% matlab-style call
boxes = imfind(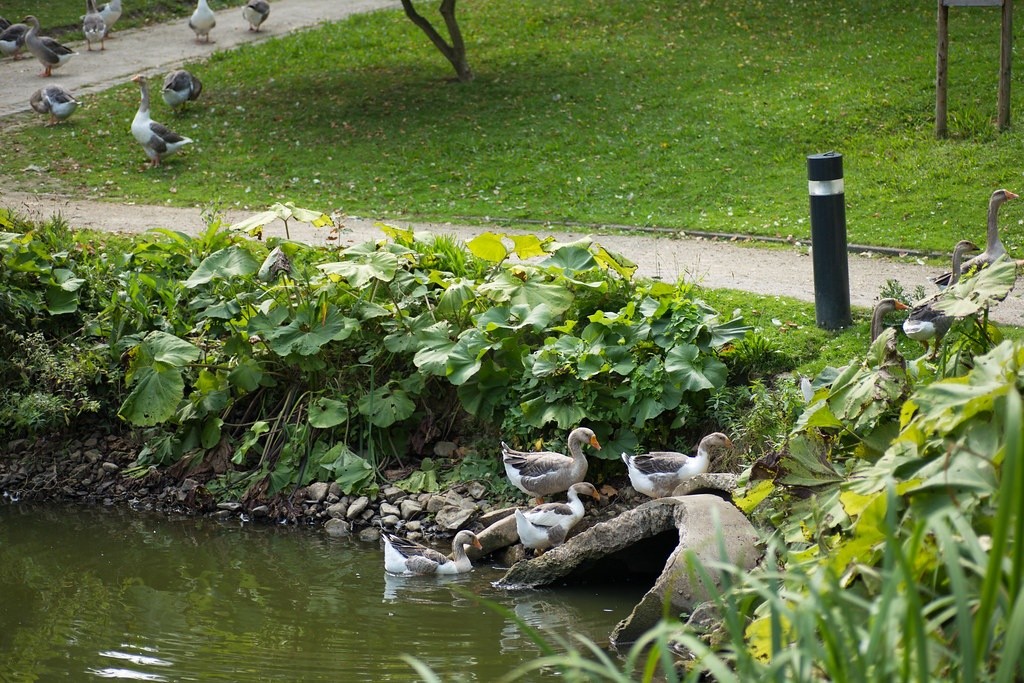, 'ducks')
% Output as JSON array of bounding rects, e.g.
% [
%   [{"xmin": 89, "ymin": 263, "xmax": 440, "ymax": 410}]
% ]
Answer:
[
  {"xmin": 904, "ymin": 239, "xmax": 981, "ymax": 360},
  {"xmin": 160, "ymin": 69, "xmax": 202, "ymax": 114},
  {"xmin": 188, "ymin": 1, "xmax": 218, "ymax": 43},
  {"xmin": 516, "ymin": 482, "xmax": 600, "ymax": 556},
  {"xmin": 871, "ymin": 298, "xmax": 911, "ymax": 371},
  {"xmin": 130, "ymin": 74, "xmax": 193, "ymax": 170},
  {"xmin": 19, "ymin": 14, "xmax": 78, "ymax": 79},
  {"xmin": 381, "ymin": 530, "xmax": 482, "ymax": 574},
  {"xmin": 929, "ymin": 188, "xmax": 1019, "ymax": 290},
  {"xmin": 500, "ymin": 426, "xmax": 601, "ymax": 507},
  {"xmin": 241, "ymin": 1, "xmax": 272, "ymax": 32},
  {"xmin": 29, "ymin": 83, "xmax": 81, "ymax": 128},
  {"xmin": 83, "ymin": 0, "xmax": 108, "ymax": 51},
  {"xmin": 79, "ymin": 0, "xmax": 123, "ymax": 41},
  {"xmin": 1, "ymin": 18, "xmax": 29, "ymax": 62},
  {"xmin": 622, "ymin": 433, "xmax": 731, "ymax": 497}
]
